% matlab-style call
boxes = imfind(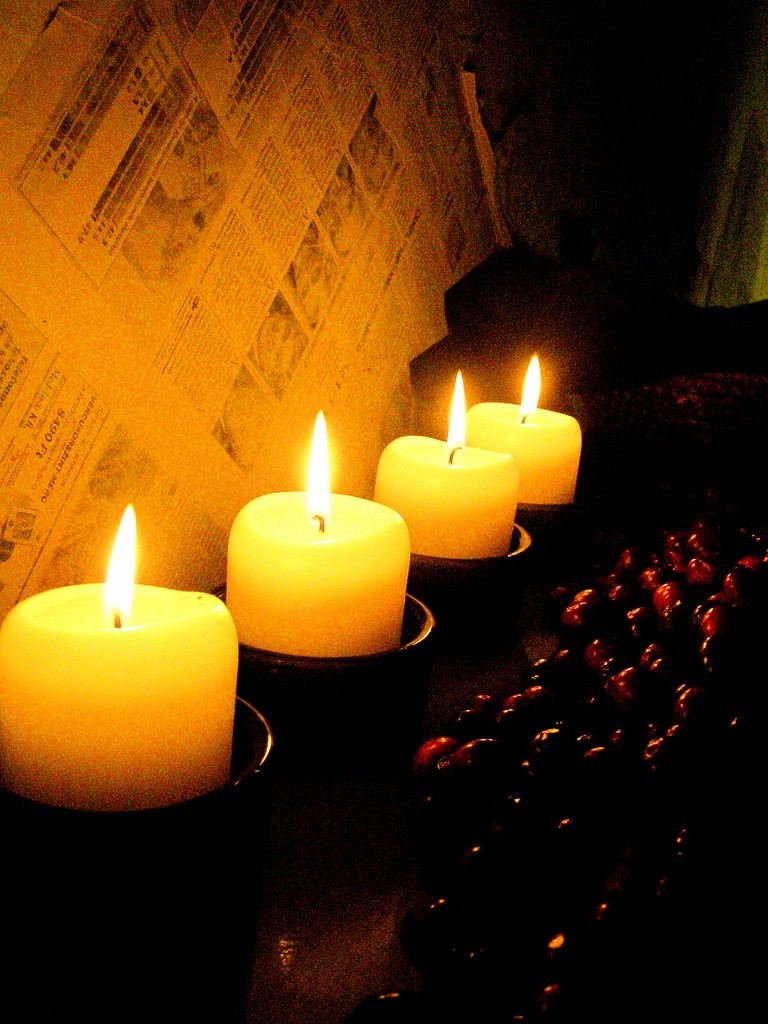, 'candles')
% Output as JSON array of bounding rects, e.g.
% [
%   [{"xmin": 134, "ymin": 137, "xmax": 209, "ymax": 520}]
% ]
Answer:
[
  {"xmin": 0, "ymin": 505, "xmax": 238, "ymax": 811},
  {"xmin": 374, "ymin": 369, "xmax": 520, "ymax": 560},
  {"xmin": 466, "ymin": 355, "xmax": 583, "ymax": 505},
  {"xmin": 227, "ymin": 408, "xmax": 412, "ymax": 657}
]
